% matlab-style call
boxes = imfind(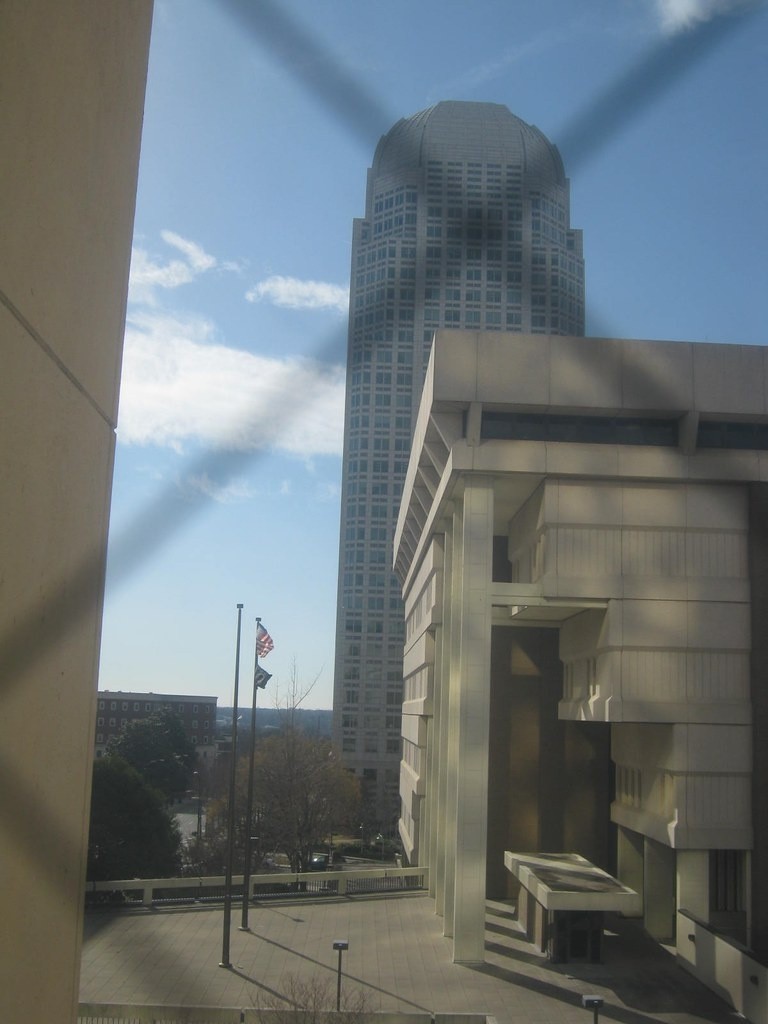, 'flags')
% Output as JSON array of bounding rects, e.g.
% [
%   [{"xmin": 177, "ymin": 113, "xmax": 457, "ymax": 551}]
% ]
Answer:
[
  {"xmin": 255, "ymin": 622, "xmax": 274, "ymax": 659},
  {"xmin": 253, "ymin": 664, "xmax": 273, "ymax": 690}
]
